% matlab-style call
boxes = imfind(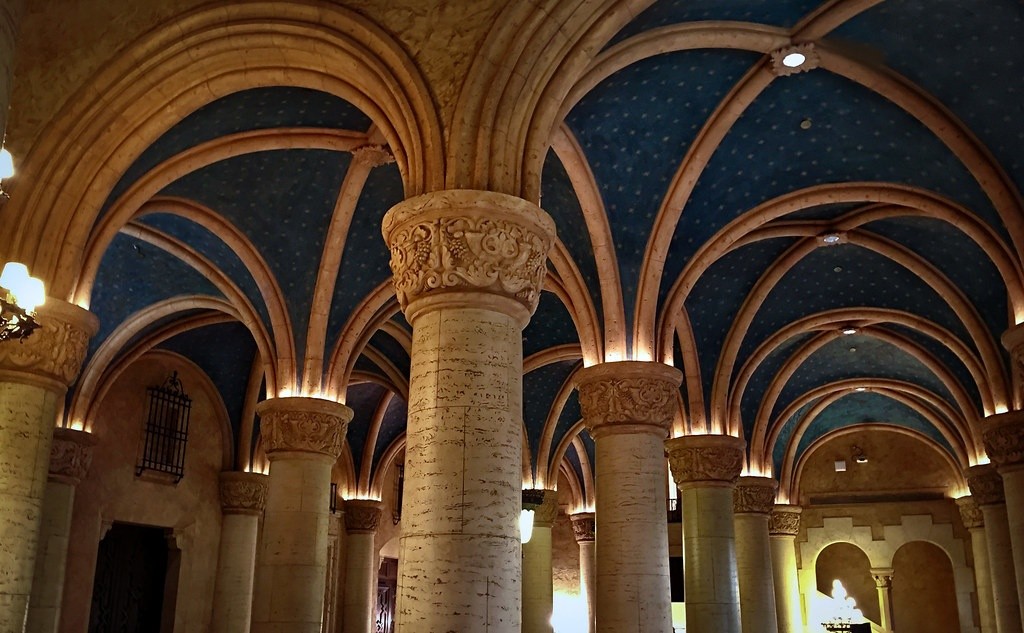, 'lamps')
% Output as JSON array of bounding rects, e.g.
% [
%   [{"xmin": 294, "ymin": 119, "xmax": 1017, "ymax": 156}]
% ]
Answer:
[
  {"xmin": 0, "ymin": 143, "xmax": 21, "ymax": 206},
  {"xmin": 0, "ymin": 256, "xmax": 47, "ymax": 344}
]
